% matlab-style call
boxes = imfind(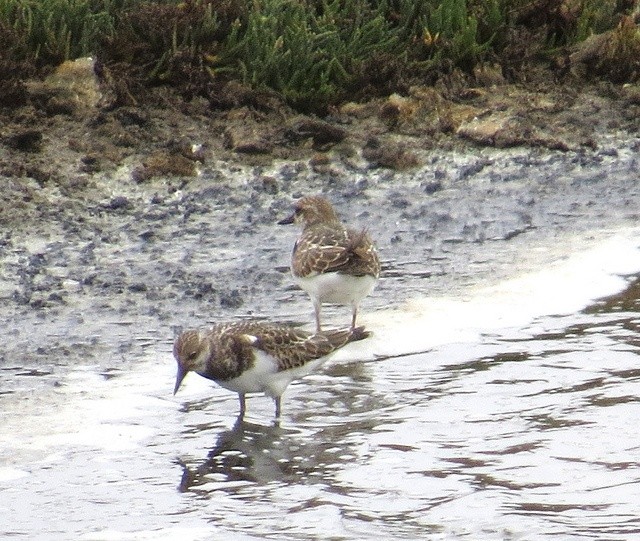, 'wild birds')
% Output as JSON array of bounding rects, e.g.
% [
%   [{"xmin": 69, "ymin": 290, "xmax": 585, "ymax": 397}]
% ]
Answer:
[
  {"xmin": 173, "ymin": 318, "xmax": 372, "ymax": 417},
  {"xmin": 278, "ymin": 196, "xmax": 379, "ymax": 332}
]
